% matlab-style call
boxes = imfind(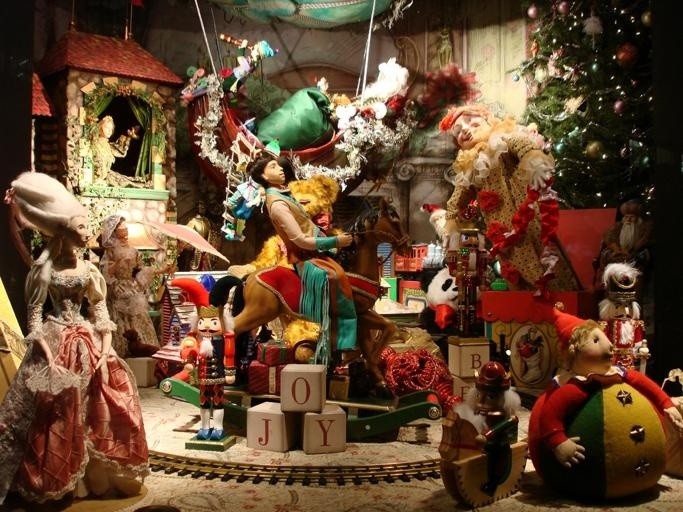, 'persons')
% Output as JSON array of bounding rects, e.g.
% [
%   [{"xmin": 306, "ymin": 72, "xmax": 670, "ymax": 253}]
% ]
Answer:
[
  {"xmin": 83, "ymin": 33, "xmax": 682, "ymax": 511},
  {"xmin": 81, "ymin": 32, "xmax": 682, "ymax": 511},
  {"xmin": 0, "ymin": 170, "xmax": 148, "ymax": 507}
]
